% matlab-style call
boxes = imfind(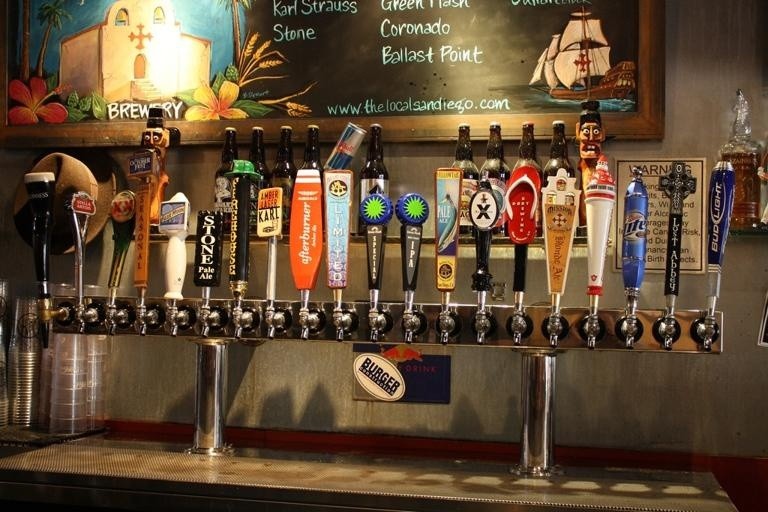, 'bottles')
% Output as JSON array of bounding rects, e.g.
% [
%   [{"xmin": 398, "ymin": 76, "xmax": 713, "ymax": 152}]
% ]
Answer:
[
  {"xmin": 358, "ymin": 125, "xmax": 390, "ymax": 236},
  {"xmin": 214, "ymin": 127, "xmax": 241, "ymax": 234},
  {"xmin": 268, "ymin": 126, "xmax": 296, "ymax": 236},
  {"xmin": 297, "ymin": 124, "xmax": 324, "ymax": 237},
  {"xmin": 543, "ymin": 121, "xmax": 582, "ymax": 237},
  {"xmin": 480, "ymin": 123, "xmax": 511, "ymax": 237},
  {"xmin": 245, "ymin": 126, "xmax": 267, "ymax": 233},
  {"xmin": 449, "ymin": 124, "xmax": 479, "ymax": 236},
  {"xmin": 512, "ymin": 122, "xmax": 543, "ymax": 237},
  {"xmin": 717, "ymin": 91, "xmax": 763, "ymax": 234}
]
